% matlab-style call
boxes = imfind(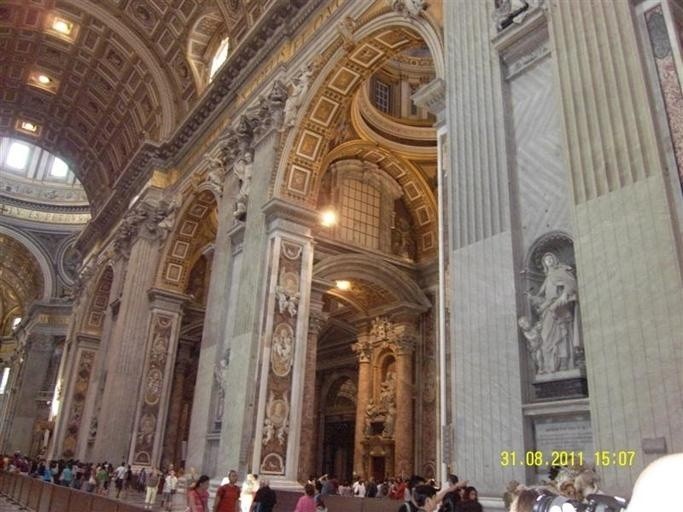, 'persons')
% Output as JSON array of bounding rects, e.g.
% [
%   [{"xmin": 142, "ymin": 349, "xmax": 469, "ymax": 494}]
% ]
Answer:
[
  {"xmin": 518, "ymin": 252, "xmax": 578, "ymax": 375},
  {"xmin": 264, "ymin": 284, "xmax": 299, "ymax": 445},
  {"xmin": 232, "ymin": 152, "xmax": 254, "ymax": 210}
]
